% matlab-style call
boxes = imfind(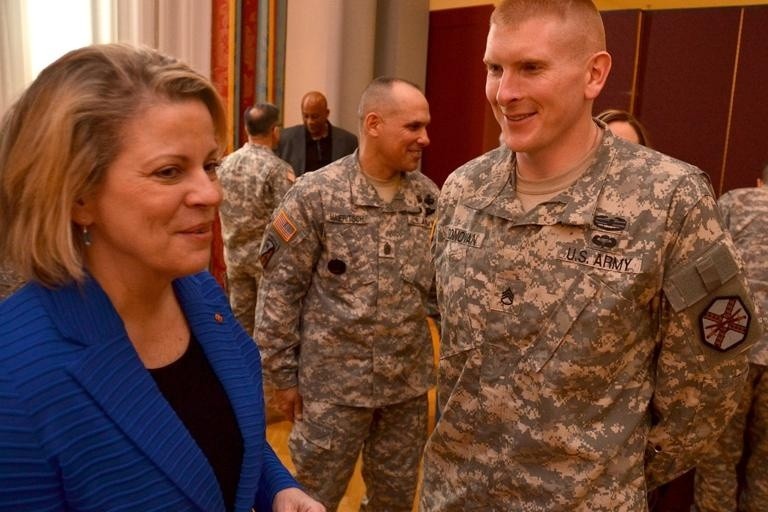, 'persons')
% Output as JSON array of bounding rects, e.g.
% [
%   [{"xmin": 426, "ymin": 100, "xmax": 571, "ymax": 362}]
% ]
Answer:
[
  {"xmin": 0, "ymin": 43, "xmax": 329, "ymax": 512},
  {"xmin": 215, "ymin": 103, "xmax": 295, "ymax": 336},
  {"xmin": 692, "ymin": 166, "xmax": 768, "ymax": 512},
  {"xmin": 595, "ymin": 110, "xmax": 657, "ymax": 151},
  {"xmin": 416, "ymin": 0, "xmax": 761, "ymax": 512},
  {"xmin": 253, "ymin": 75, "xmax": 441, "ymax": 512},
  {"xmin": 273, "ymin": 91, "xmax": 358, "ymax": 177}
]
